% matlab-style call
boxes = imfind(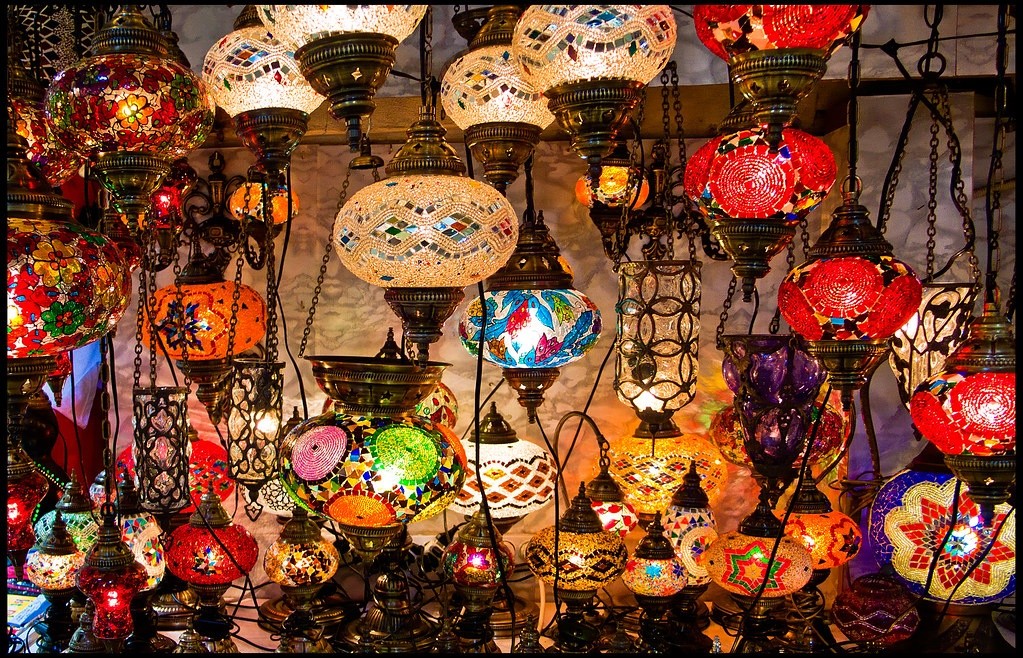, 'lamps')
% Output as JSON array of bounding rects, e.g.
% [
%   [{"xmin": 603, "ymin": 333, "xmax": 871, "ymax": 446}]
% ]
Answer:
[{"xmin": 7, "ymin": 1, "xmax": 1018, "ymax": 656}]
